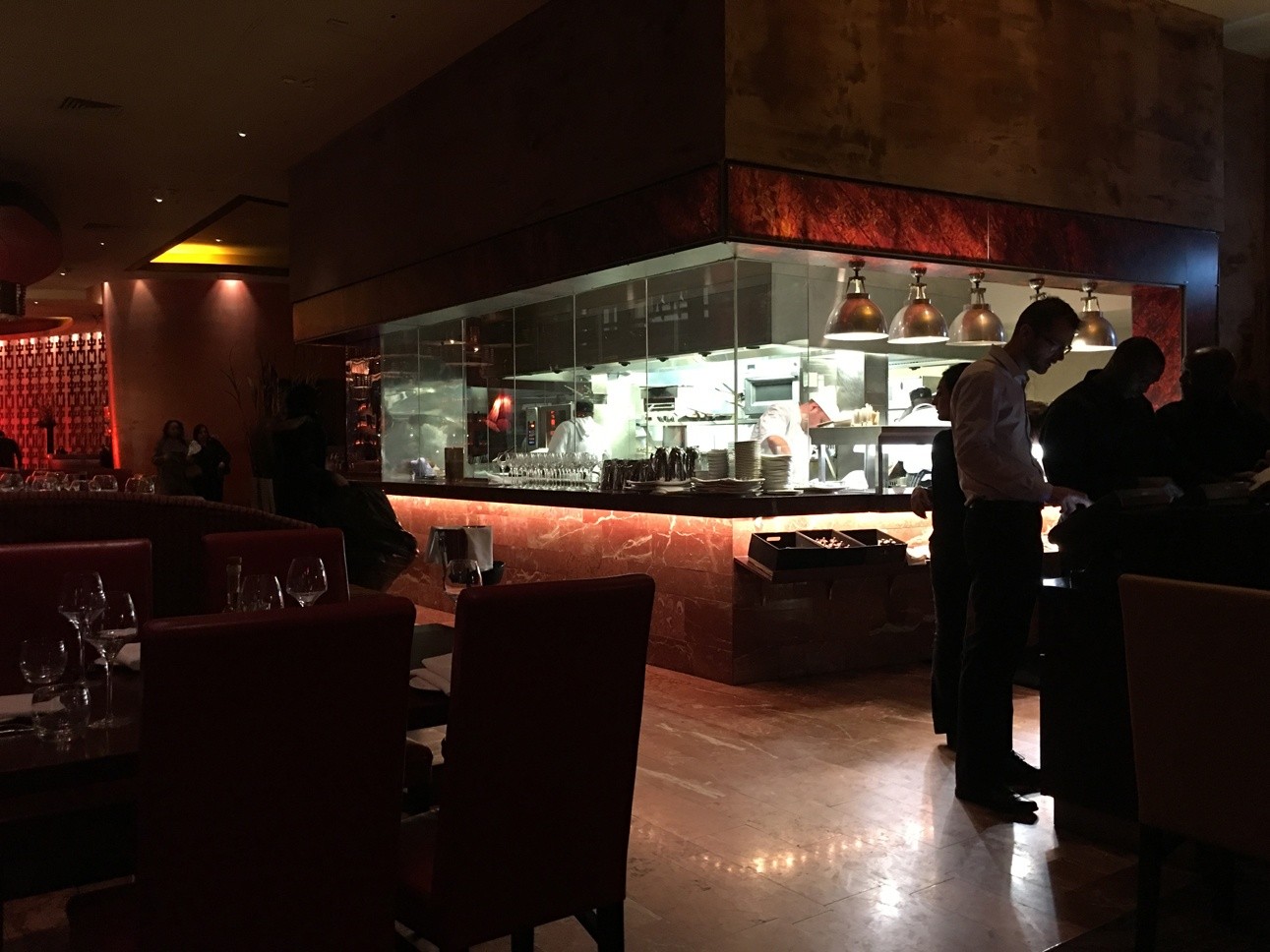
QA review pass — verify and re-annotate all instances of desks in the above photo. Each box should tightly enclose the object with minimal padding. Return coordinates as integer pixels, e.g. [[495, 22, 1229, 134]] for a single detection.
[[1, 623, 454, 797]]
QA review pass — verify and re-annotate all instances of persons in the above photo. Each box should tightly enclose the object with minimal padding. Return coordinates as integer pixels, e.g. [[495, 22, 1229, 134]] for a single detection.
[[306, 471, 417, 589], [1037, 337, 1166, 805], [1155, 346, 1266, 585], [182, 424, 231, 503], [911, 363, 1047, 753], [0, 431, 22, 468], [951, 299, 1094, 813], [152, 421, 193, 493]]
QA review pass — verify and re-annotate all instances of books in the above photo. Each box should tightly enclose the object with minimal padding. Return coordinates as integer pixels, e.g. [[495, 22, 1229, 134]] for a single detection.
[[1194, 465, 1270, 497], [1116, 477, 1175, 506]]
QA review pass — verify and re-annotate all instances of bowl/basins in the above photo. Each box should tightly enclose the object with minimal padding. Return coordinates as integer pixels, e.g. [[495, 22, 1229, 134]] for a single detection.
[[694, 441, 792, 489]]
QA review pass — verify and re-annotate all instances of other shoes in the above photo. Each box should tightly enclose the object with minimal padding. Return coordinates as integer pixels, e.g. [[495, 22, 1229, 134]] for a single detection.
[[1006, 751, 1043, 794], [956, 781, 1039, 814], [947, 728, 957, 752]]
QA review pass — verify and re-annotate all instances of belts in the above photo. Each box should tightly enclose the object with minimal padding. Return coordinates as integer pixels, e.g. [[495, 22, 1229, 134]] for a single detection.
[[967, 498, 1044, 512]]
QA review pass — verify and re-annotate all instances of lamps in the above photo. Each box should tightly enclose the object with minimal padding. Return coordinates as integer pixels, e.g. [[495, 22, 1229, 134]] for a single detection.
[[947, 272, 1008, 346], [1072, 282, 1117, 352], [824, 260, 889, 340], [888, 266, 949, 344], [484, 393, 513, 432]]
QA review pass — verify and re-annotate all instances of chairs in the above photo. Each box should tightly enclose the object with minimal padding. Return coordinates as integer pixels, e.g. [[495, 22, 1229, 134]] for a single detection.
[[394, 574, 656, 951], [65, 596, 415, 952], [201, 528, 434, 810], [1118, 573, 1270, 952], [0, 539, 154, 696]]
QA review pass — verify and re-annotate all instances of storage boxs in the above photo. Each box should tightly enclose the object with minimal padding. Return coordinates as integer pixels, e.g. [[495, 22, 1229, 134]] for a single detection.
[[747, 528, 908, 570]]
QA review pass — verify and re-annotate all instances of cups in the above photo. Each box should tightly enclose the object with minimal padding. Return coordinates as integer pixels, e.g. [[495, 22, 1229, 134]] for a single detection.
[[19, 635, 69, 684], [236, 572, 284, 613], [0, 470, 118, 492], [124, 477, 155, 494]]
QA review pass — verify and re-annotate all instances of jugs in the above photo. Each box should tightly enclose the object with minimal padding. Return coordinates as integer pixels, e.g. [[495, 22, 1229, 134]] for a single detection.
[[599, 445, 700, 492]]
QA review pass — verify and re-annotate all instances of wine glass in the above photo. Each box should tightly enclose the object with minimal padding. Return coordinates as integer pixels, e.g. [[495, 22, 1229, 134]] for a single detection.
[[80, 590, 139, 730], [57, 570, 107, 707], [498, 452, 599, 493], [286, 557, 328, 608], [32, 681, 92, 764], [443, 558, 483, 605]]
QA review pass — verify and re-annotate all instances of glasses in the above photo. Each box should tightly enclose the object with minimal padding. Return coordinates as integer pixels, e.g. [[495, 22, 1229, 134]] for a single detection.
[[1027, 323, 1071, 356]]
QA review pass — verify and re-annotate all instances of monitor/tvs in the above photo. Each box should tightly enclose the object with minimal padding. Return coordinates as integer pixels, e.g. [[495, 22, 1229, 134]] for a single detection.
[[1048, 486, 1176, 544], [1191, 481, 1252, 511]]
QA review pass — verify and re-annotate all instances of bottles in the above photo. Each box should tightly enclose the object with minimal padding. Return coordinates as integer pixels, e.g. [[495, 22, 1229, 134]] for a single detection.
[[221, 555, 244, 615], [133, 473, 144, 493]]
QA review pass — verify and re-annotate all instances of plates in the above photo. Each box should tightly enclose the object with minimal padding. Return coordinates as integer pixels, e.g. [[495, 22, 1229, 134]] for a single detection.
[[409, 677, 442, 691], [623, 477, 847, 494]]
